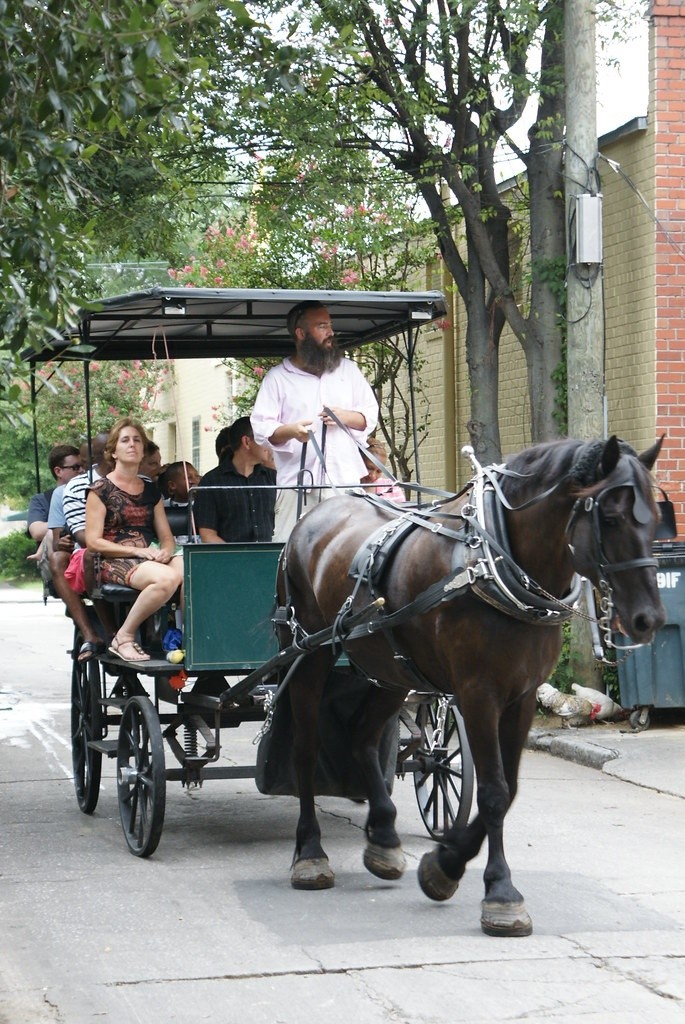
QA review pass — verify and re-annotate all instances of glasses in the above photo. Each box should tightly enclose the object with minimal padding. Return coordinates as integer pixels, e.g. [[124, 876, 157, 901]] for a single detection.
[[60, 463, 84, 471]]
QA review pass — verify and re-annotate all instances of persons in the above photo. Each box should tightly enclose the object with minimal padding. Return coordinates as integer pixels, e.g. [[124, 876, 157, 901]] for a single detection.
[[23, 416, 408, 666], [249, 301, 379, 544]]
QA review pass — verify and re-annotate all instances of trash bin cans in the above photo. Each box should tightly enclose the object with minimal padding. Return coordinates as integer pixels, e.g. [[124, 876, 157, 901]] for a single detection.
[[610, 554, 684, 731]]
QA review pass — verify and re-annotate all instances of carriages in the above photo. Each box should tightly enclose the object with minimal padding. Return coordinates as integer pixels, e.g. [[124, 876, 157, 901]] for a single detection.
[[19, 284, 676, 937]]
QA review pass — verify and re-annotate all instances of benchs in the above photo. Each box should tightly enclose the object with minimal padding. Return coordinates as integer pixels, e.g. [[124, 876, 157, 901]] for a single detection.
[[90, 548, 139, 605]]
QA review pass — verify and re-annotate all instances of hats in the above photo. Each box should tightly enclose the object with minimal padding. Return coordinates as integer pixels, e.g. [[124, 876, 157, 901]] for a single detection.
[[286, 300, 322, 338]]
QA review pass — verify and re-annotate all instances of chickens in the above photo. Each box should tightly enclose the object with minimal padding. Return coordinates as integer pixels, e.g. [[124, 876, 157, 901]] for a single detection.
[[570, 683, 626, 727], [535, 682, 602, 732]]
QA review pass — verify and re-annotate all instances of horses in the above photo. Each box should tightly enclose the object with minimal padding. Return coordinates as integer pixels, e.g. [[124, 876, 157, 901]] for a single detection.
[[272, 432, 669, 938]]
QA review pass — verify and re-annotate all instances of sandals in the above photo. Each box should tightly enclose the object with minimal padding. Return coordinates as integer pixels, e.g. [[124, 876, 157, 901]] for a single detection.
[[109, 635, 150, 661]]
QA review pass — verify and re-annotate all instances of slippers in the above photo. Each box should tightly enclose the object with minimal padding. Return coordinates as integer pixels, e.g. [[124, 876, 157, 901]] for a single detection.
[[80, 641, 106, 663]]
